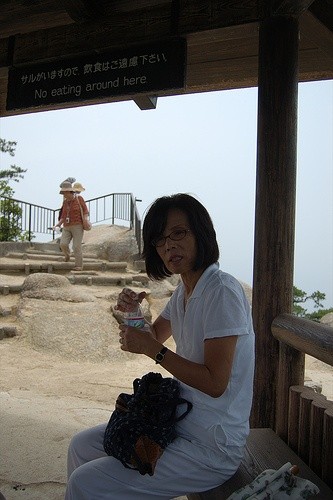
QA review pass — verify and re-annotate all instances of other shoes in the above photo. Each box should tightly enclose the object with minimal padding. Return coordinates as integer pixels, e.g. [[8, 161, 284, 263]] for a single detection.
[[73, 267, 82, 271], [64, 257, 70, 262]]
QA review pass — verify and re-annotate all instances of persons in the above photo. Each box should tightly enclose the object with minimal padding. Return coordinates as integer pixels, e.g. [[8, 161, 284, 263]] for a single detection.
[[53, 178, 92, 270], [66, 193, 255, 500]]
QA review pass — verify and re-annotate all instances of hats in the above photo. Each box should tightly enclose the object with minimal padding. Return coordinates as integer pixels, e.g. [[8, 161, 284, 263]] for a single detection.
[[73, 183, 85, 192], [60, 182, 75, 194]]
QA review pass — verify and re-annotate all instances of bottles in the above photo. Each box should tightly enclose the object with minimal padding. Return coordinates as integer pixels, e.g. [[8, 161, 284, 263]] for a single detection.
[[52, 226, 62, 233]]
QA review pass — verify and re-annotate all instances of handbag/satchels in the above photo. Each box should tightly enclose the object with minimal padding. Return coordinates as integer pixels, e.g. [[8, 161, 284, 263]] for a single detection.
[[104, 372, 193, 476], [82, 218, 92, 231]]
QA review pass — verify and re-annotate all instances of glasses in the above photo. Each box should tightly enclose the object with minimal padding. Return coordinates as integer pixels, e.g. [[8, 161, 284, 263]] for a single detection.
[[152, 229, 191, 247]]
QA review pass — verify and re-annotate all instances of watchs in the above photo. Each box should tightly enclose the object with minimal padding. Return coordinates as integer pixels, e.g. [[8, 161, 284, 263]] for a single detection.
[[155, 347, 168, 364]]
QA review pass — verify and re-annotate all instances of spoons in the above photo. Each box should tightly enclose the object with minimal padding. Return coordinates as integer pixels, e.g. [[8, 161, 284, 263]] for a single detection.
[[125, 284, 153, 326]]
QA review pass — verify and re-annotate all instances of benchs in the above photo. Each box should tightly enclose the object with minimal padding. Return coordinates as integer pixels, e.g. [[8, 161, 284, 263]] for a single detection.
[[188, 428, 333, 500]]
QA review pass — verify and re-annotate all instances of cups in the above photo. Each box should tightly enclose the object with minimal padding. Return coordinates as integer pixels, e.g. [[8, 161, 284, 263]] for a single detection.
[[122, 316, 145, 329]]
